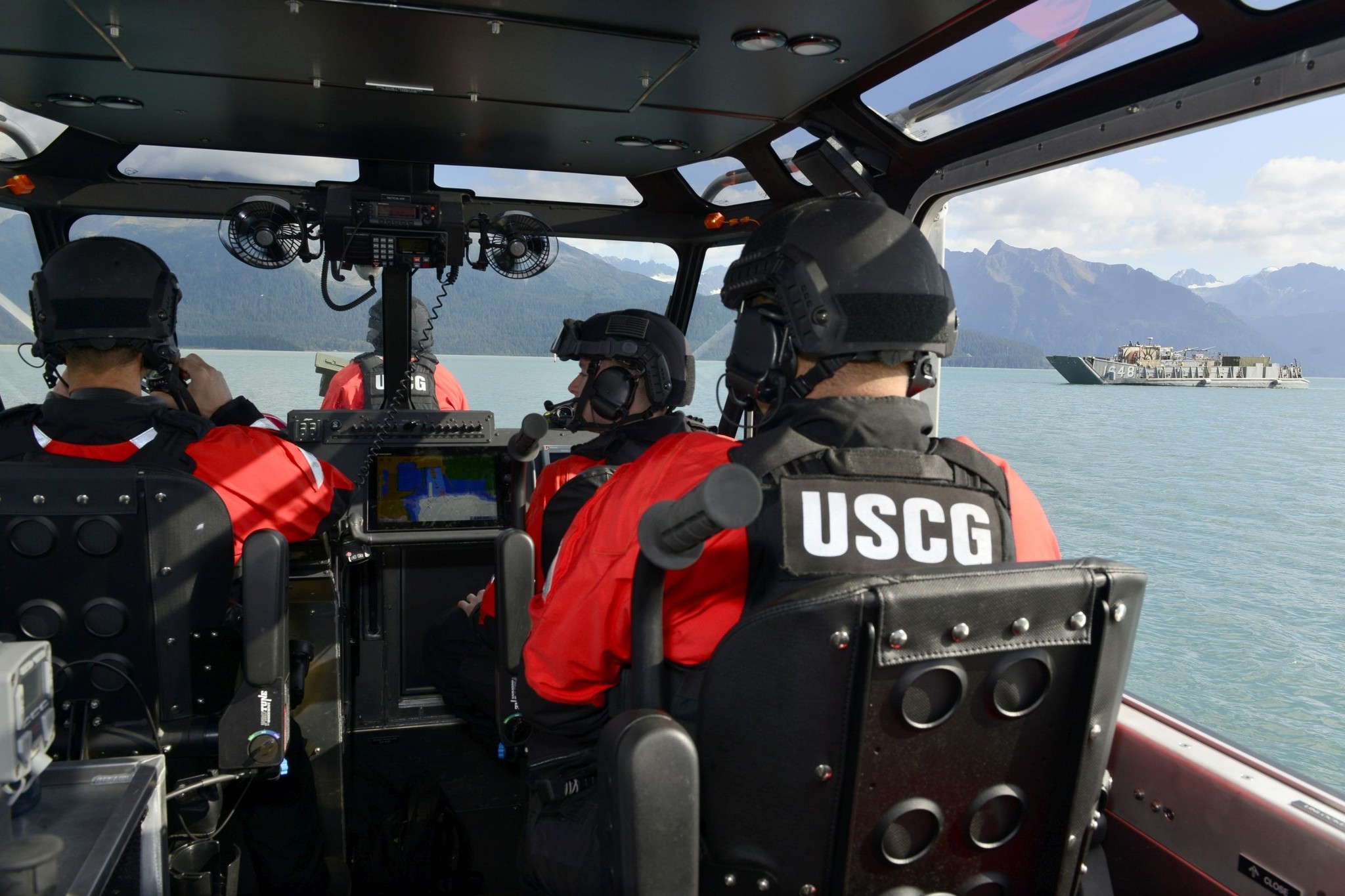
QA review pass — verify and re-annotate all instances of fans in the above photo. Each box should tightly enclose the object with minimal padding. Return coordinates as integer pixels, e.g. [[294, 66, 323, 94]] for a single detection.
[[218, 194, 304, 268], [479, 209, 560, 279]]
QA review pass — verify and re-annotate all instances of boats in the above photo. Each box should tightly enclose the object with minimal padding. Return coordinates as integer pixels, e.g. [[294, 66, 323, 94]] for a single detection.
[[1045, 337, 1310, 389]]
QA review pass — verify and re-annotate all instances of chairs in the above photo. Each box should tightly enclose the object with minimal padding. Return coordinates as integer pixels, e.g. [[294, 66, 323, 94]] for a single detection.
[[495, 465, 619, 761], [605, 554, 1149, 896], [0, 461, 290, 782]]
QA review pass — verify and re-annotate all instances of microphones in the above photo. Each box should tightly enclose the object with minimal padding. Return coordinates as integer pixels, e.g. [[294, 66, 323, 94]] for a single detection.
[[543, 396, 580, 411]]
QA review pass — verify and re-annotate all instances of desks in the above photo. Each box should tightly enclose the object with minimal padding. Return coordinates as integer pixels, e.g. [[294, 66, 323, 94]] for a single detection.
[[0, 754, 167, 896]]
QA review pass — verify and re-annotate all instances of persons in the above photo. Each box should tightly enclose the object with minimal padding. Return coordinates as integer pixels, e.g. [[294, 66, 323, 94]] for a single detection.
[[319, 298, 467, 414], [0, 237, 340, 896], [514, 187, 1065, 896], [434, 309, 723, 764]]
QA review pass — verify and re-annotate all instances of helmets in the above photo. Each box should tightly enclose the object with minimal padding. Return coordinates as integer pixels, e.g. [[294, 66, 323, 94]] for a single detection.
[[551, 309, 694, 406], [719, 191, 960, 361], [27, 237, 181, 355], [367, 294, 433, 350]]
